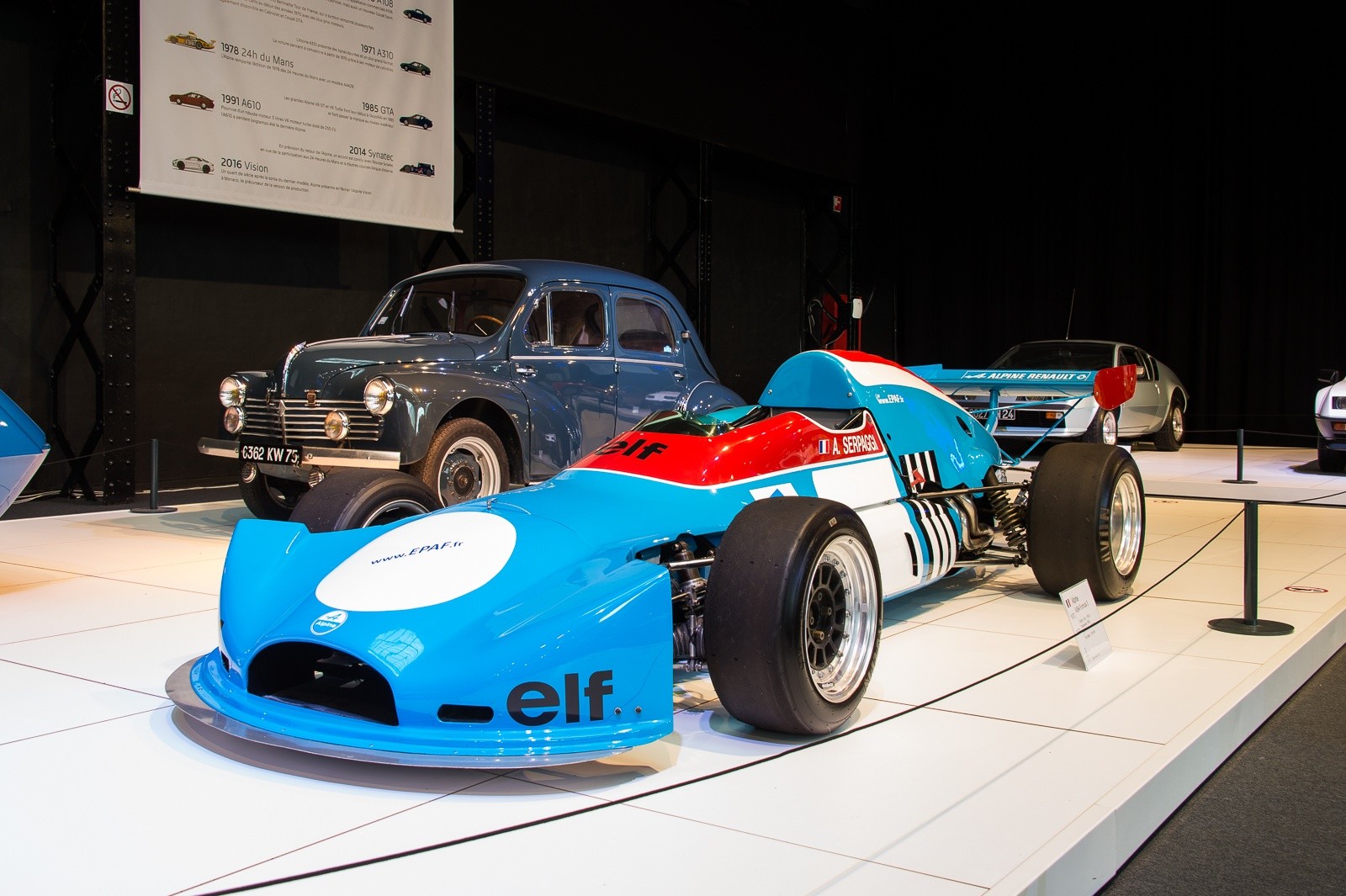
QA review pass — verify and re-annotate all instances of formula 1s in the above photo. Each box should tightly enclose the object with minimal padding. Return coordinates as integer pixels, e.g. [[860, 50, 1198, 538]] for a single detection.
[[162, 332, 1147, 768]]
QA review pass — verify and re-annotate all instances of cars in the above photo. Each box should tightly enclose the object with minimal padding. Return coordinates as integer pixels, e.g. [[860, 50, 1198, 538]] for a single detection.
[[400, 162, 435, 177], [404, 8, 431, 23], [1312, 369, 1346, 470], [955, 340, 1187, 451], [173, 156, 214, 175], [198, 260, 751, 522], [169, 92, 215, 110], [400, 114, 432, 130], [400, 61, 430, 76], [165, 30, 217, 50]]
